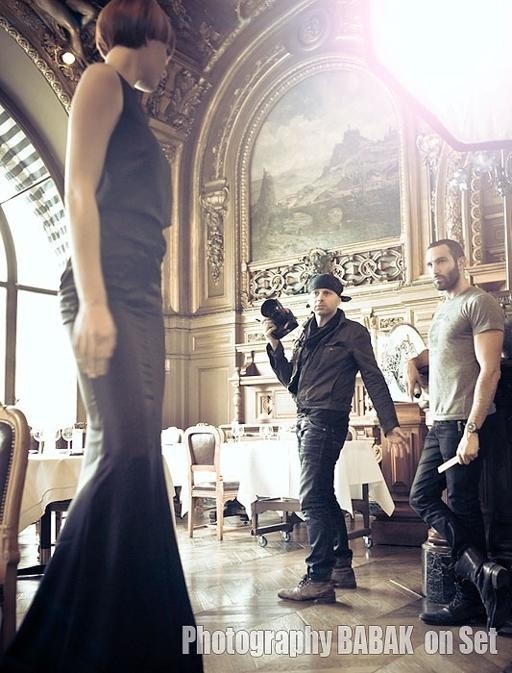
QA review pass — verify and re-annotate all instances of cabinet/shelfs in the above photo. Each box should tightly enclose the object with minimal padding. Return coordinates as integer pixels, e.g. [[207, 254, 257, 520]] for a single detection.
[[373, 403, 435, 547]]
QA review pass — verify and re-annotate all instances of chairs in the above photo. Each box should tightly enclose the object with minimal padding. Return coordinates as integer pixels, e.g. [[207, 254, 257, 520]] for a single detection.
[[0, 402, 30, 656]]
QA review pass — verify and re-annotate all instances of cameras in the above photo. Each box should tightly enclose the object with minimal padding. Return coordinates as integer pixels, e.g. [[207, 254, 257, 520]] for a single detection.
[[260, 298, 299, 339]]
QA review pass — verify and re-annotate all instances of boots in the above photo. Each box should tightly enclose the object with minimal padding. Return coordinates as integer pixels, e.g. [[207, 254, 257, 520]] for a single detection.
[[419, 548, 510, 631]]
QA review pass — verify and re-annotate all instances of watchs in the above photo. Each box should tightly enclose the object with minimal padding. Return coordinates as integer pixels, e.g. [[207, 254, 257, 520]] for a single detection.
[[463, 423, 478, 434]]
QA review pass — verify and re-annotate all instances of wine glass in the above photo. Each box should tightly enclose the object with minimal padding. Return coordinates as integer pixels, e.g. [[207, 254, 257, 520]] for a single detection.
[[32, 424, 75, 452], [231, 424, 291, 443]]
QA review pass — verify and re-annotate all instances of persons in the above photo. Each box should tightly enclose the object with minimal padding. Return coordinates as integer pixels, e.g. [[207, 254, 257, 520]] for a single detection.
[[408, 239, 509, 635], [260, 276, 406, 606], [0, 1, 207, 673]]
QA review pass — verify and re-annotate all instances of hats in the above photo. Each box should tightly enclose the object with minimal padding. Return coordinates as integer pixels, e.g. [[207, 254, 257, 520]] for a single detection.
[[308, 274, 352, 301]]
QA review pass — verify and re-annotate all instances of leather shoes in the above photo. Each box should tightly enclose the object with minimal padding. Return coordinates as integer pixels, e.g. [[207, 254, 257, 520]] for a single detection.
[[277, 567, 355, 603]]
